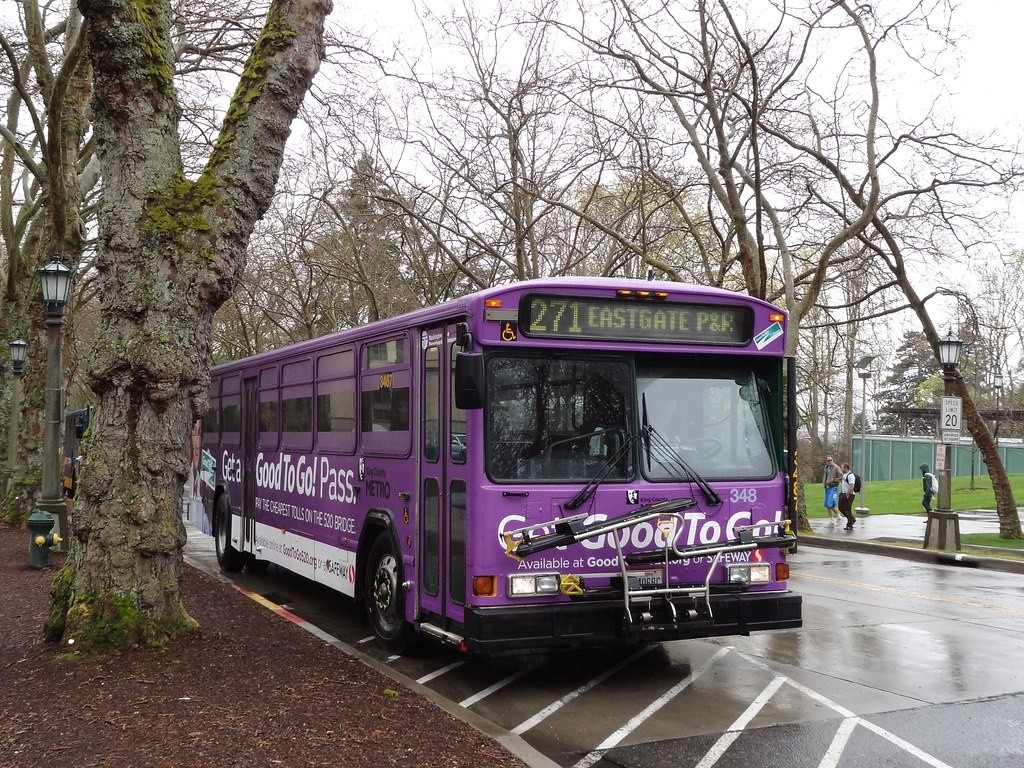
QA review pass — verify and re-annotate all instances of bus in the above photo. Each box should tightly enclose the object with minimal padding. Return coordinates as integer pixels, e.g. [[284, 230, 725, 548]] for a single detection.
[[185, 274, 803, 662]]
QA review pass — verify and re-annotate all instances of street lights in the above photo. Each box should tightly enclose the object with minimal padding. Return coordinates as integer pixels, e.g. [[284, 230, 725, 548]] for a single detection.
[[854, 355, 876, 514], [36, 249, 72, 556], [934, 326, 965, 511]]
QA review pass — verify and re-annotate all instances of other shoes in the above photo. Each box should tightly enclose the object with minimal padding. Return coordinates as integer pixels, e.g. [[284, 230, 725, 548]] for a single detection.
[[836, 516, 844, 526], [847, 517, 856, 526], [824, 522, 835, 528], [924, 520, 928, 522], [844, 526, 853, 530]]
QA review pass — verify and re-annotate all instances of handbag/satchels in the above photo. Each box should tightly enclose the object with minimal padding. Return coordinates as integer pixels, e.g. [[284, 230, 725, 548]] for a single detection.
[[925, 473, 938, 494]]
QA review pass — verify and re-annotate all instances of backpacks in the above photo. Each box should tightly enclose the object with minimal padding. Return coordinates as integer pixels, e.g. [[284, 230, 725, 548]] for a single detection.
[[846, 472, 861, 493]]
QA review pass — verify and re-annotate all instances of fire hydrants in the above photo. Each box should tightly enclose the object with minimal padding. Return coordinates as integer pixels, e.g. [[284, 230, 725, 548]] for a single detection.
[[26, 511, 65, 572]]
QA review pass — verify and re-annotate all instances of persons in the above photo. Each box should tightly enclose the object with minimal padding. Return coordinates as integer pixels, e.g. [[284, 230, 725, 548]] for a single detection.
[[838, 463, 856, 530], [189, 414, 212, 535], [648, 399, 677, 446], [919, 463, 934, 523], [823, 455, 844, 527]]
